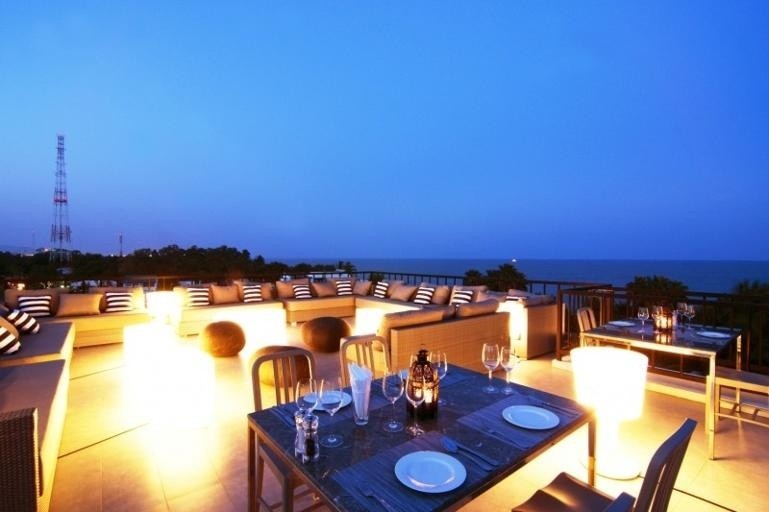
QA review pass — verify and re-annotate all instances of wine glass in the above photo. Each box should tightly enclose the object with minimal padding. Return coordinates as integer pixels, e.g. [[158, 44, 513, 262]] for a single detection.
[[428, 350, 449, 408], [480, 340, 500, 396], [319, 376, 347, 449], [651, 305, 664, 335], [381, 369, 406, 434], [404, 374, 428, 437], [676, 301, 687, 328], [409, 349, 428, 368], [500, 344, 519, 397], [684, 305, 697, 331], [637, 307, 649, 332], [294, 376, 319, 412]]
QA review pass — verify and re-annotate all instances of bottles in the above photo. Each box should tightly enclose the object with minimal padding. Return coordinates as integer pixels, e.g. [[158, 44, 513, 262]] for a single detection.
[[292, 405, 321, 466]]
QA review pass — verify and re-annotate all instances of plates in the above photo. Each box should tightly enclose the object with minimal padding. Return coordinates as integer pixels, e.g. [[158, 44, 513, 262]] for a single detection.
[[609, 320, 635, 327], [303, 388, 353, 409], [697, 330, 731, 338], [394, 449, 467, 493], [397, 367, 409, 381], [499, 404, 562, 432]]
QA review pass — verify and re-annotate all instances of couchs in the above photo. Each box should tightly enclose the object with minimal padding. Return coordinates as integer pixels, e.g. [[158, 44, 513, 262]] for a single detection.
[[0, 358, 69, 512], [352, 299, 513, 384]]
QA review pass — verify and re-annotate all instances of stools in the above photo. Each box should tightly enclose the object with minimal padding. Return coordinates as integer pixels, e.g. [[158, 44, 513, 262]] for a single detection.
[[704, 365, 769, 462]]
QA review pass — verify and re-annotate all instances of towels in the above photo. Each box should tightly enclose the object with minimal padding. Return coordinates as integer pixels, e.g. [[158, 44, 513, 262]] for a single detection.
[[347, 364, 374, 420]]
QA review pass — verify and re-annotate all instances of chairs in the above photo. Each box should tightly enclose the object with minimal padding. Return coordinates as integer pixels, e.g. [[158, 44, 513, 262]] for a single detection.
[[248, 344, 339, 511], [598, 490, 640, 511], [509, 416, 699, 510], [575, 305, 599, 348], [338, 335, 393, 391]]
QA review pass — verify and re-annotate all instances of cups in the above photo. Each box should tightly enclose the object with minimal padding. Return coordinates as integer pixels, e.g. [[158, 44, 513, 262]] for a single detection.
[[349, 376, 373, 426]]
